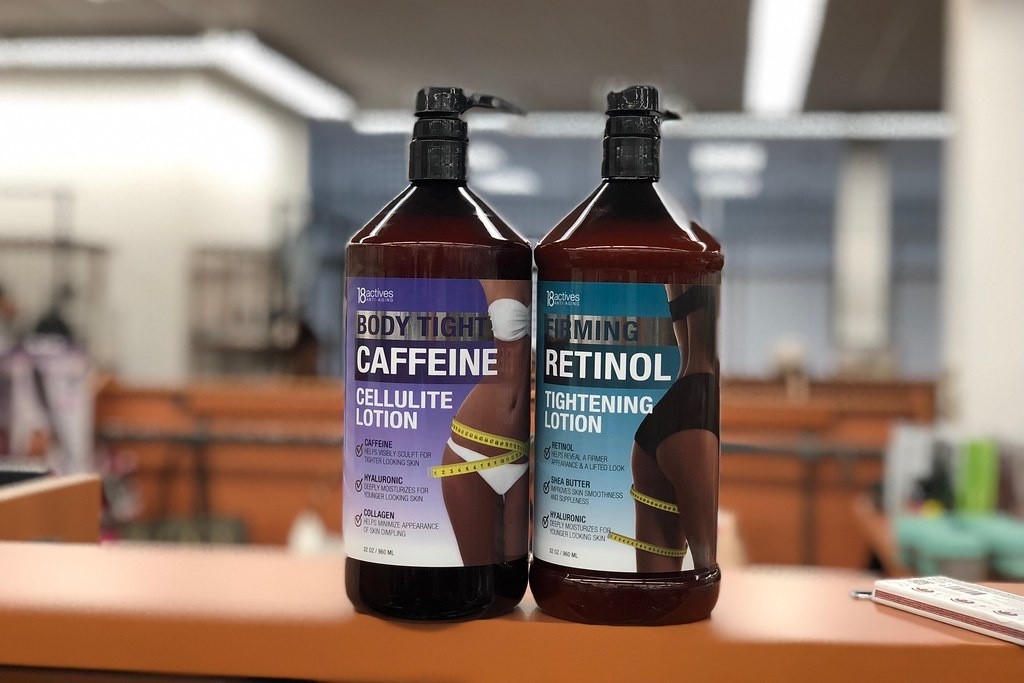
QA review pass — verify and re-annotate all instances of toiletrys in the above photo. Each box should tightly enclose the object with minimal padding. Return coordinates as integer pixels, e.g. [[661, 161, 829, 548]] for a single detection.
[[341, 86, 532, 626], [530, 82, 724, 624]]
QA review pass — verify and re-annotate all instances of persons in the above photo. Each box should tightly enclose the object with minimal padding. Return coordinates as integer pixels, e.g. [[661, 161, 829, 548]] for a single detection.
[[440, 280, 527, 565], [0, 286, 105, 484], [631, 283, 716, 574]]
